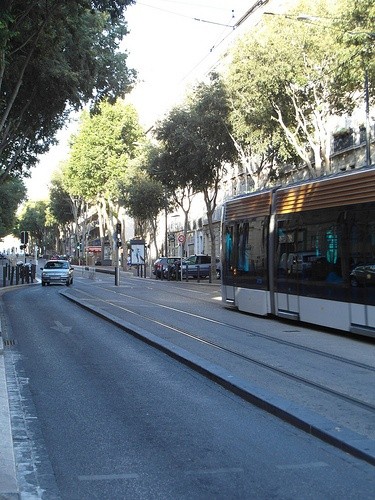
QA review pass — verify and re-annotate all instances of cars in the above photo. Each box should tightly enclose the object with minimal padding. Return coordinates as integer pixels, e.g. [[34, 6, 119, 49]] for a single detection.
[[49, 252, 70, 259], [349, 265, 375, 287], [40, 260, 75, 286]]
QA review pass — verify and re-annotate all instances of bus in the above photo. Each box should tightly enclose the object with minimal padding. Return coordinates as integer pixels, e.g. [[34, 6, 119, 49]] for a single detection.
[[219, 164, 375, 339]]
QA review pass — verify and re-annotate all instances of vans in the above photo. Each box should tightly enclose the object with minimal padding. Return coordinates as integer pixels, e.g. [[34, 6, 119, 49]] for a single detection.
[[279, 252, 322, 279]]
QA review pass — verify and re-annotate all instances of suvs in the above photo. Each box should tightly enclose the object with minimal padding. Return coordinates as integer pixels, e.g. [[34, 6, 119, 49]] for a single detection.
[[153, 254, 221, 281]]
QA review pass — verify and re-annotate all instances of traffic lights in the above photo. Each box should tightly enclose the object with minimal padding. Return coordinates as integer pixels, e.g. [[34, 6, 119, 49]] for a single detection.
[[21, 231, 29, 244]]
